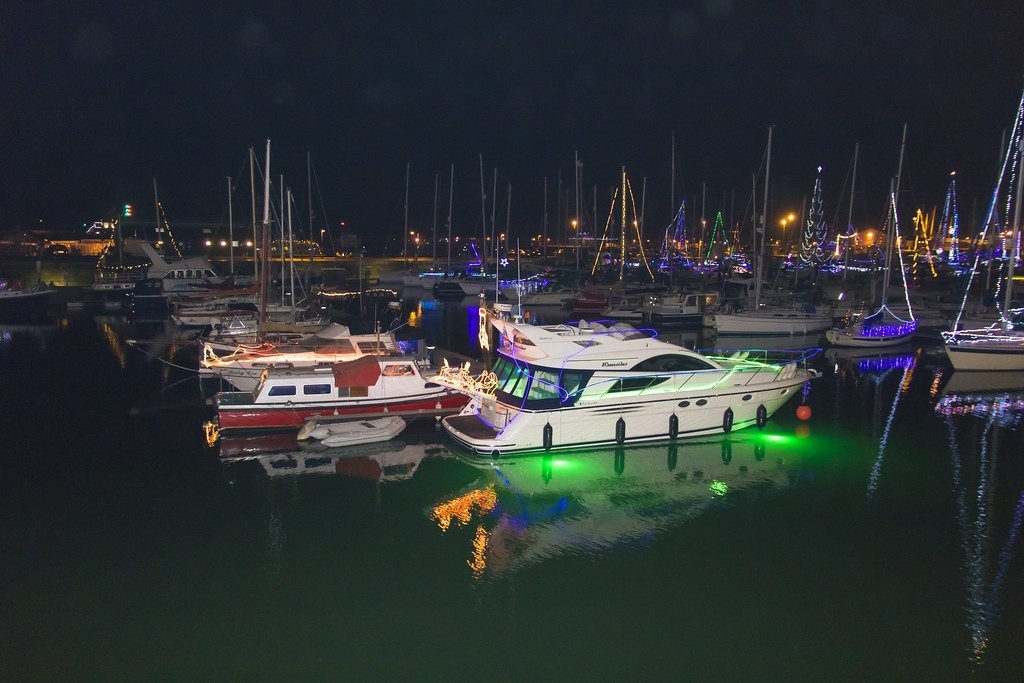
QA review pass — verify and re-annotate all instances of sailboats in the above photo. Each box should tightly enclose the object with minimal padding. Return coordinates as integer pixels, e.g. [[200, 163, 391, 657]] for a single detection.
[[934, 371, 1024, 531], [91, 97, 1024, 371]]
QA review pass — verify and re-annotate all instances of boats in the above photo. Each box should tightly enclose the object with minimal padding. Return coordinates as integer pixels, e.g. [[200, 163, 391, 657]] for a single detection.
[[202, 320, 481, 448], [219, 436, 457, 508], [443, 239, 823, 456]]
